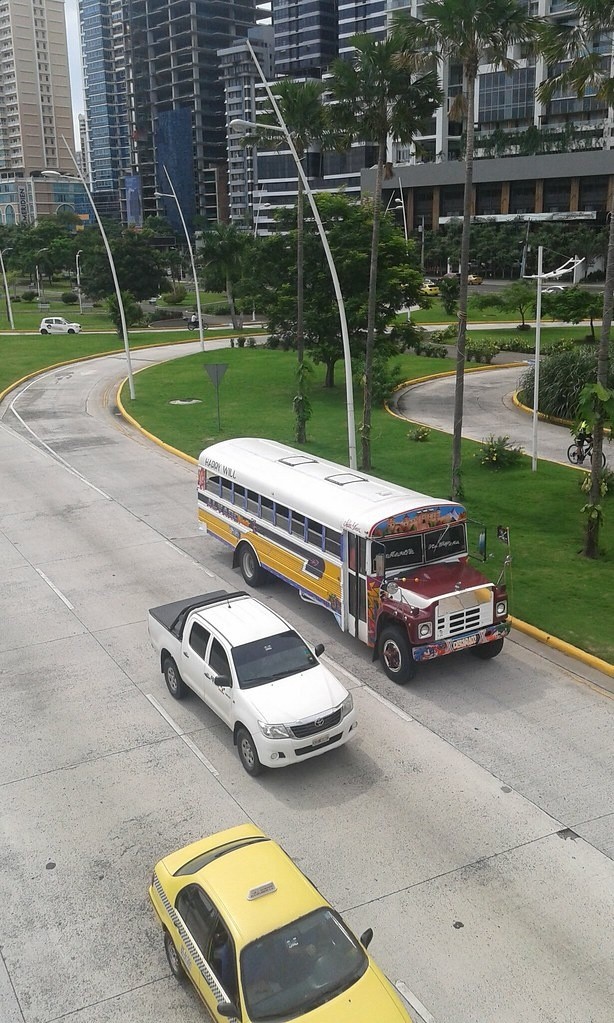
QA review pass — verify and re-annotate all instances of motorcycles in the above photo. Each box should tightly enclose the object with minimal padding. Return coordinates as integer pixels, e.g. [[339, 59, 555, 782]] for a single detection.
[[187, 319, 209, 331]]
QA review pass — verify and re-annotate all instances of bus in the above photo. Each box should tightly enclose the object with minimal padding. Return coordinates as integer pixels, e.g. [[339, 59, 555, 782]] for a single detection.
[[196, 437, 513, 684]]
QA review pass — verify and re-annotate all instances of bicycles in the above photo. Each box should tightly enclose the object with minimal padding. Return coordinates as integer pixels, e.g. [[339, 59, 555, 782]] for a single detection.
[[567, 441, 606, 469]]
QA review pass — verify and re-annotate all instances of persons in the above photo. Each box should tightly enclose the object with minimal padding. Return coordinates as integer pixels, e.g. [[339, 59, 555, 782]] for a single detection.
[[191, 313, 198, 328], [576, 420, 593, 465]]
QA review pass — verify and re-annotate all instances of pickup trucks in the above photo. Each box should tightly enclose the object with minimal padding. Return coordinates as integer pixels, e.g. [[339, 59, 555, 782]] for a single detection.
[[147, 590, 360, 778]]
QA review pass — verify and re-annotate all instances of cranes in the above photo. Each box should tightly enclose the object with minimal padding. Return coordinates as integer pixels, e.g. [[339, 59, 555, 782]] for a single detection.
[[443, 273, 460, 285]]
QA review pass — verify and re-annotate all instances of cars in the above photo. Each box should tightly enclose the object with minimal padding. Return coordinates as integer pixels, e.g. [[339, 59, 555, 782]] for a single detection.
[[148, 294, 162, 305], [400, 276, 445, 291], [148, 823, 414, 1023], [541, 285, 570, 295], [467, 274, 483, 286], [417, 281, 440, 297], [39, 317, 82, 336]]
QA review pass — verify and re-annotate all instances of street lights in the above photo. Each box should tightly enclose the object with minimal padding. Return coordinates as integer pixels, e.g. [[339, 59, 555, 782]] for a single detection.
[[36, 248, 49, 304], [153, 165, 205, 352], [229, 38, 357, 472], [41, 133, 136, 401], [0, 248, 16, 331], [75, 250, 84, 314], [395, 176, 410, 322]]
